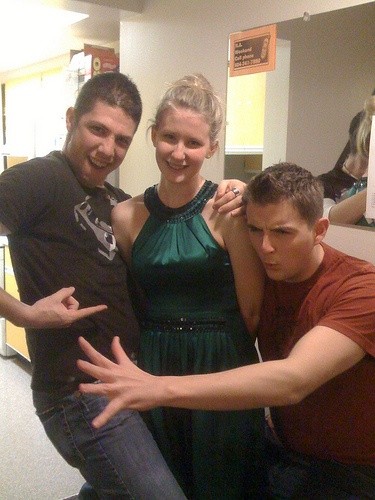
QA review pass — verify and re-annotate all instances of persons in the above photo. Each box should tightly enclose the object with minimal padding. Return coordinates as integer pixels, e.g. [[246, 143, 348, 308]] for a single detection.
[[317, 88, 375, 230], [76, 163, 375, 500], [0, 72, 250, 500], [110, 72, 266, 500]]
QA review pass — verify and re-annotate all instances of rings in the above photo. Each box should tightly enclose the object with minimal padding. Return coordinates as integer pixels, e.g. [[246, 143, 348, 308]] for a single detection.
[[232, 186, 241, 198]]
